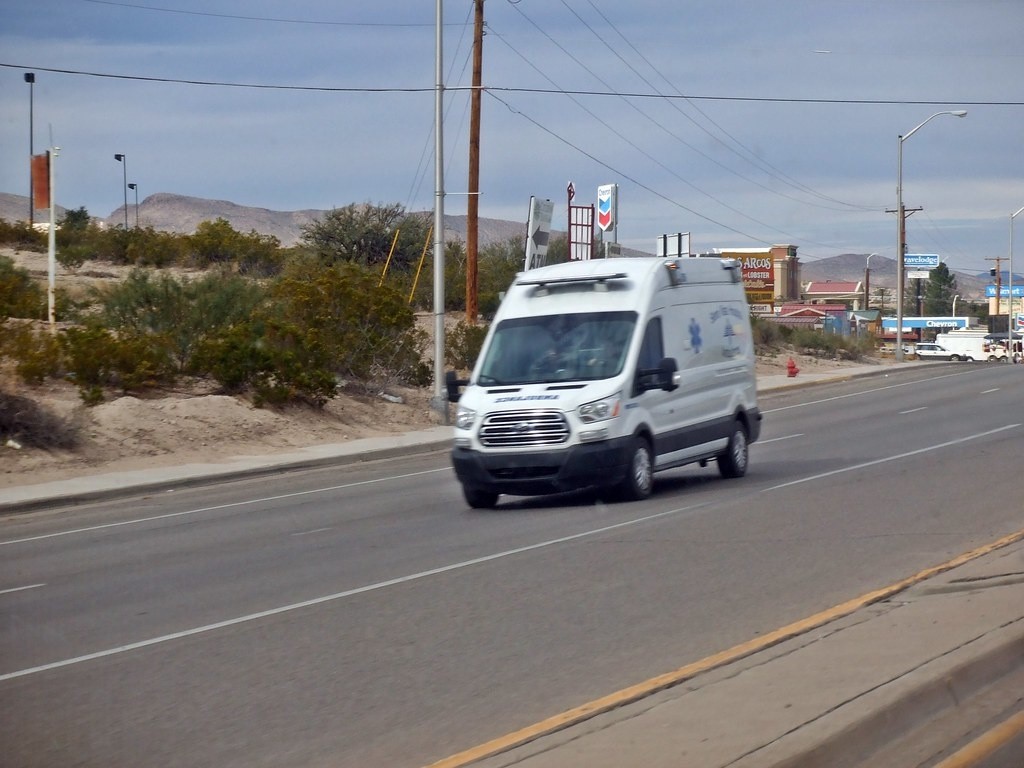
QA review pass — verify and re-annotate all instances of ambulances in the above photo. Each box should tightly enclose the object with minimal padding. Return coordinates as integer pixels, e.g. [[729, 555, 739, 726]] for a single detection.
[[444, 257, 763, 509]]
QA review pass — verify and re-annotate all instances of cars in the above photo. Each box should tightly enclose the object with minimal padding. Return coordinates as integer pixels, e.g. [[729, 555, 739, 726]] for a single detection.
[[915, 342, 967, 362], [875, 347, 887, 353], [889, 347, 897, 355], [904, 346, 917, 355]]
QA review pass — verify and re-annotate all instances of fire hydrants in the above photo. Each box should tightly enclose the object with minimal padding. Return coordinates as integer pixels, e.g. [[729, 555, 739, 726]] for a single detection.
[[787, 357, 800, 378]]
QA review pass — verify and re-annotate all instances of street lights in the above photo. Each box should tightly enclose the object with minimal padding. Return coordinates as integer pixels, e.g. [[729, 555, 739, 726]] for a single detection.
[[23, 71, 35, 230], [128, 182, 139, 230], [115, 154, 128, 232], [879, 288, 886, 316], [865, 253, 878, 310], [897, 110, 966, 355], [952, 294, 959, 329]]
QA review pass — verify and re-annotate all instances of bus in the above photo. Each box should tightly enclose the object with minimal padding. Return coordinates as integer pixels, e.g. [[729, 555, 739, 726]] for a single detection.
[[935, 333, 993, 362]]
